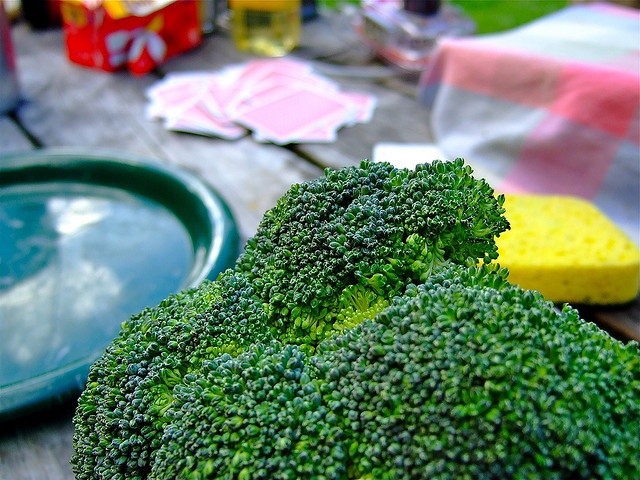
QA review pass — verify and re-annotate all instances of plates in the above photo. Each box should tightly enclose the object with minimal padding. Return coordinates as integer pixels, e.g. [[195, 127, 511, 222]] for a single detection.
[[1, 152, 240, 417]]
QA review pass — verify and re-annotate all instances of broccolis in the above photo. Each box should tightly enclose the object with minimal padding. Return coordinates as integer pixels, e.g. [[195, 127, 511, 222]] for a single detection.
[[68, 154, 640, 479]]
